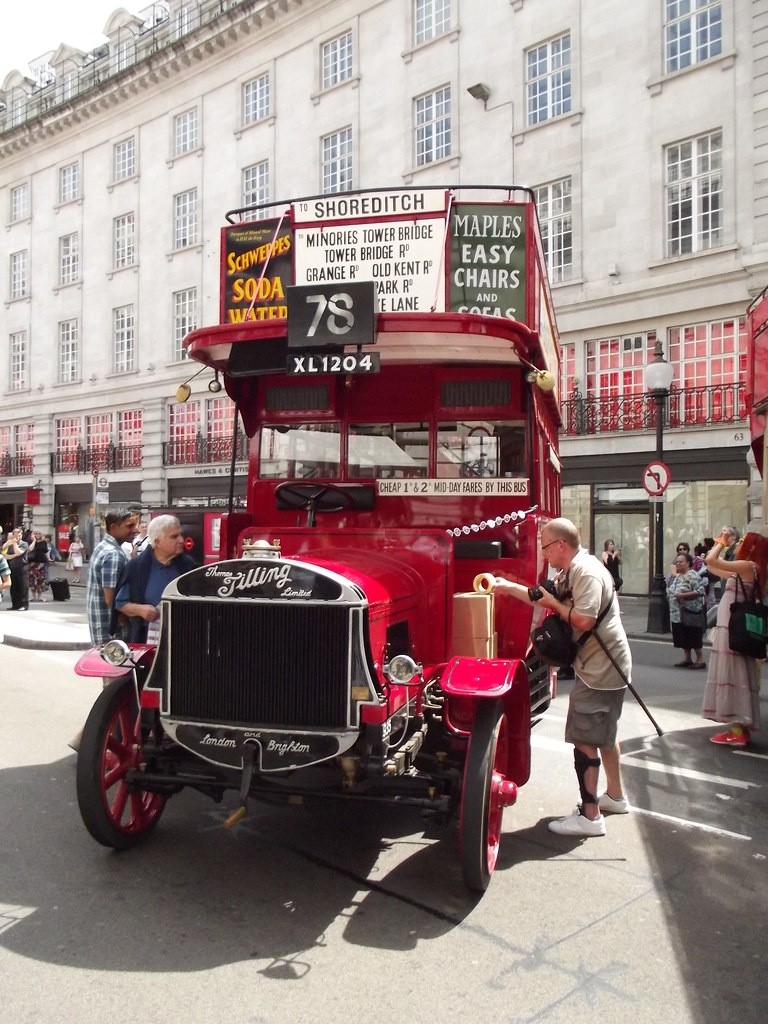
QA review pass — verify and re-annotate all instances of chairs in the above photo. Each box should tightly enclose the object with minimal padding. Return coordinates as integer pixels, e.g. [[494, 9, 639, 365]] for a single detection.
[[272, 482, 381, 529]]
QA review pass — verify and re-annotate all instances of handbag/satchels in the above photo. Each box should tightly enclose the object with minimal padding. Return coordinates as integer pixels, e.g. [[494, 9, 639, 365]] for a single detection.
[[531, 615, 576, 669], [728, 561, 768, 659], [66, 559, 74, 571], [616, 578, 623, 586], [679, 606, 705, 628]]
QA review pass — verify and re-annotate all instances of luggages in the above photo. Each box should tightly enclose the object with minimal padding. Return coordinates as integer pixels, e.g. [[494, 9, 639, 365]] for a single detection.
[[51, 577, 70, 601]]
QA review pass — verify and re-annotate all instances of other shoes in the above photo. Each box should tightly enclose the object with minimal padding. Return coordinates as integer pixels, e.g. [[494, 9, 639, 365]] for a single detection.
[[71, 580, 76, 583], [69, 731, 82, 751], [30, 598, 37, 601], [7, 607, 15, 610], [18, 607, 26, 611], [75, 580, 80, 583], [674, 661, 693, 667], [38, 597, 46, 602], [688, 662, 705, 669]]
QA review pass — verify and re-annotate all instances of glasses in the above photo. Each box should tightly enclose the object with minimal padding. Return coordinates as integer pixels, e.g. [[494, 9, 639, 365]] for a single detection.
[[679, 548, 686, 551], [541, 539, 566, 551], [739, 538, 744, 544]]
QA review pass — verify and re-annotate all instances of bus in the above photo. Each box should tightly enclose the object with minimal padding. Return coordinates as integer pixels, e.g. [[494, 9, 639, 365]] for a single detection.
[[70, 184, 574, 895]]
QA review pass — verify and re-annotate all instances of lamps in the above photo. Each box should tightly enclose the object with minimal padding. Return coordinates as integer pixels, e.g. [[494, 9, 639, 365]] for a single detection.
[[608, 264, 619, 277], [467, 82, 492, 100]]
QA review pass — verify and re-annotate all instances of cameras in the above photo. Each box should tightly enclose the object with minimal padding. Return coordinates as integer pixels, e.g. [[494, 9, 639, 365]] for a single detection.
[[527, 580, 557, 602]]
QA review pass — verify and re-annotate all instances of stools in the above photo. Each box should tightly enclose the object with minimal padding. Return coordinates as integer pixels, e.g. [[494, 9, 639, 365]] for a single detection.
[[454, 540, 503, 560]]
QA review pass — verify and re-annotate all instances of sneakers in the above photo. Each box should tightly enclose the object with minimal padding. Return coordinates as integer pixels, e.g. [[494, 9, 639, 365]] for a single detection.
[[710, 730, 747, 746], [599, 792, 628, 814], [549, 802, 606, 836]]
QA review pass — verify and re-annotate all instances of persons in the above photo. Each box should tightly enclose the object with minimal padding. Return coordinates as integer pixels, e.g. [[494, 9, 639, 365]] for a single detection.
[[720, 526, 740, 600], [68, 508, 142, 753], [121, 535, 139, 565], [115, 514, 200, 644], [132, 521, 151, 557], [68, 535, 85, 583], [0, 524, 62, 611], [491, 517, 632, 836], [665, 537, 720, 670], [706, 528, 768, 746], [602, 539, 625, 615]]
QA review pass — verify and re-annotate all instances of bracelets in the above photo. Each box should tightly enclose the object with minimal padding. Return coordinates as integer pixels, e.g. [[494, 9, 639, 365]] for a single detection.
[[714, 537, 729, 547]]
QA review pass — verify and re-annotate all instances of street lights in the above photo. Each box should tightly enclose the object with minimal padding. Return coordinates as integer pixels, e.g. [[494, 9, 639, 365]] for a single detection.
[[641, 339, 671, 634]]
